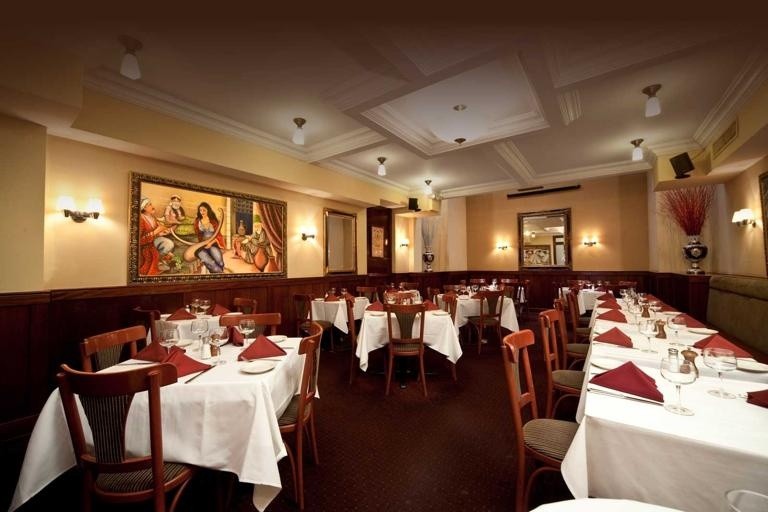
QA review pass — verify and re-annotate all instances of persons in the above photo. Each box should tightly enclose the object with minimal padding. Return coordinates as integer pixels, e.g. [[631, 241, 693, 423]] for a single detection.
[[161, 194, 187, 224], [193, 201, 227, 274], [241, 213, 275, 264], [139, 197, 174, 271]]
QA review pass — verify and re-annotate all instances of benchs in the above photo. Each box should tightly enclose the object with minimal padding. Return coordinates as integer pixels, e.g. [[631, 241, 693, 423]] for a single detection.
[[702, 275, 768, 365]]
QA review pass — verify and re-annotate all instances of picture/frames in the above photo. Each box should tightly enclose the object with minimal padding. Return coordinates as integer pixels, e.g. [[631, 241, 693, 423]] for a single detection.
[[127, 171, 287, 287], [759, 170, 768, 276], [523, 244, 551, 266]]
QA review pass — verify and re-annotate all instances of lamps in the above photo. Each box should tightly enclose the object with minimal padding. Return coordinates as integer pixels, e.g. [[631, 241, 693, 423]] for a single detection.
[[732, 208, 755, 227], [584, 232, 596, 246], [498, 240, 509, 249], [57, 196, 104, 223], [377, 157, 386, 176], [118, 35, 143, 81], [631, 139, 643, 160], [400, 238, 409, 247], [430, 105, 488, 145], [642, 84, 661, 117], [424, 180, 432, 193], [291, 117, 305, 145]]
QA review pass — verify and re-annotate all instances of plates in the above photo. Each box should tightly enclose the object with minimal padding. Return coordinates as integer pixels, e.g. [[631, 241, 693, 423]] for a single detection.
[[735, 358, 767, 372], [267, 335, 287, 343], [590, 358, 626, 370], [688, 327, 719, 335], [239, 361, 275, 374], [173, 338, 192, 346]]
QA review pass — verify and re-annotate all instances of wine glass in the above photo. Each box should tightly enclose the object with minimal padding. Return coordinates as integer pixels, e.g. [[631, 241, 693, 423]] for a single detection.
[[210, 329, 229, 364], [191, 321, 209, 350], [191, 299, 211, 319], [639, 321, 659, 354], [575, 278, 610, 291], [660, 358, 698, 417], [324, 287, 349, 300], [238, 320, 256, 348], [619, 286, 683, 321], [702, 348, 735, 400], [667, 316, 686, 346], [159, 327, 179, 354]]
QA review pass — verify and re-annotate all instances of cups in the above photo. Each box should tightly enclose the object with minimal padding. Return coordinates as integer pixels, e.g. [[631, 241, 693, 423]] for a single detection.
[[455, 278, 499, 298], [390, 281, 409, 292]]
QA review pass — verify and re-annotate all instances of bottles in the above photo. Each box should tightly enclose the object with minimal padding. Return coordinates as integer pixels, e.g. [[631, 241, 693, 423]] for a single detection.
[[667, 348, 680, 372], [185, 304, 190, 314], [680, 348, 699, 378]]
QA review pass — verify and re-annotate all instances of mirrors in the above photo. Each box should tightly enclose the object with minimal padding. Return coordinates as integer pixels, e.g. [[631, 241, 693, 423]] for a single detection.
[[323, 207, 357, 273], [517, 208, 571, 273]]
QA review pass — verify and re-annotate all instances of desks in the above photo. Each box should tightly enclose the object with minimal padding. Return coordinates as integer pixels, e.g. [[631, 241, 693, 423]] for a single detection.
[[559, 291, 768, 512]]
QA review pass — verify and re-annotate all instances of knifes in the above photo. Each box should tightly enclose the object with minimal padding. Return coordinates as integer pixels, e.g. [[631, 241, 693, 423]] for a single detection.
[[184, 363, 217, 385]]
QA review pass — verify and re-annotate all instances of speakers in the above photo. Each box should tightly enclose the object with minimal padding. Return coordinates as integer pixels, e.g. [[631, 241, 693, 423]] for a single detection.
[[670, 151, 695, 176], [408, 198, 417, 210]]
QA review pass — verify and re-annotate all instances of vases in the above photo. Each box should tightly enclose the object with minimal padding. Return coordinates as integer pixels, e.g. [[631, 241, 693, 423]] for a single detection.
[[683, 235, 707, 275], [422, 246, 434, 273]]
